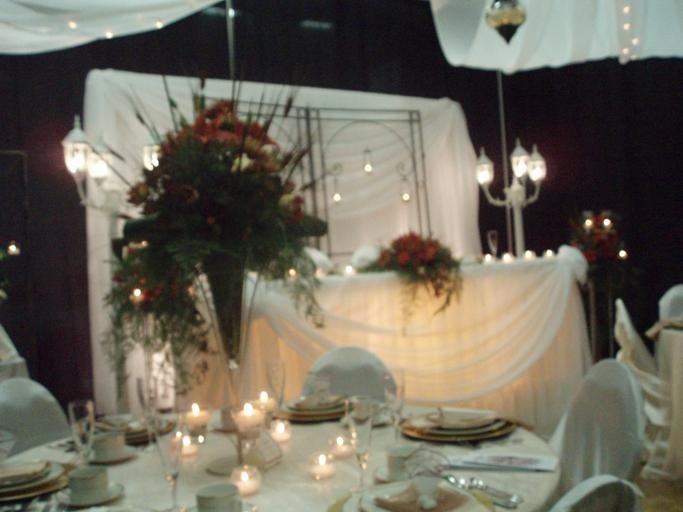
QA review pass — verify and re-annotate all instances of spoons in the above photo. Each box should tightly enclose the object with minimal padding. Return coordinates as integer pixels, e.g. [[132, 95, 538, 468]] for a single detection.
[[447, 474, 517, 509], [470, 475, 524, 503]]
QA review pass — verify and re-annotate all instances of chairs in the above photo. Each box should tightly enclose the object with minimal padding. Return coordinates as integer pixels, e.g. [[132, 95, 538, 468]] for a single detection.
[[614, 299, 666, 478], [549, 359, 646, 503], [552, 474, 639, 512], [1, 377, 69, 457], [301, 346, 397, 405], [660, 285, 682, 320]]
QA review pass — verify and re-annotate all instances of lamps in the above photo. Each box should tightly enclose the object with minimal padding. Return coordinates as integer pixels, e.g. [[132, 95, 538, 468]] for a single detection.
[[486, 0, 526, 43]]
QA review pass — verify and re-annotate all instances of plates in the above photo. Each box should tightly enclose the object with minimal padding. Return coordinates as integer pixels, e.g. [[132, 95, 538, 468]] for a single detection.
[[86, 412, 155, 434], [373, 464, 390, 482], [327, 480, 496, 512], [406, 410, 506, 436], [56, 483, 126, 507], [358, 479, 479, 511], [125, 417, 177, 444], [0, 472, 70, 503], [399, 417, 517, 443], [0, 463, 72, 492], [273, 404, 348, 422], [1, 460, 49, 487], [280, 396, 351, 416], [91, 426, 152, 438], [79, 446, 136, 463], [184, 501, 260, 512]]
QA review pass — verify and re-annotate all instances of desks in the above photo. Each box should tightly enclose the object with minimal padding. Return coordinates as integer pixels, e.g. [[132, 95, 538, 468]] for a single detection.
[[656, 316, 682, 478], [172, 257, 589, 433], [0, 404, 563, 512]]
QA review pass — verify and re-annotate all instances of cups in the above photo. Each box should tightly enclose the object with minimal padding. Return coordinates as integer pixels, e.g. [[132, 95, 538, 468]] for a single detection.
[[385, 446, 416, 474], [195, 484, 241, 512], [90, 431, 125, 456], [69, 467, 109, 501]]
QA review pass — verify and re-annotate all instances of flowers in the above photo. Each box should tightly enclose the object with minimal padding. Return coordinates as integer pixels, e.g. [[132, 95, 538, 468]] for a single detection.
[[357, 228, 464, 318], [564, 208, 628, 274], [83, 49, 343, 395]]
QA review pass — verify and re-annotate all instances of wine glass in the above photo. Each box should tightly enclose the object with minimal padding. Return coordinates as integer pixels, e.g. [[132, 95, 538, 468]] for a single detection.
[[487, 228, 499, 263], [346, 395, 375, 491], [154, 407, 190, 512], [68, 400, 95, 466], [267, 361, 287, 406], [383, 366, 408, 441]]
